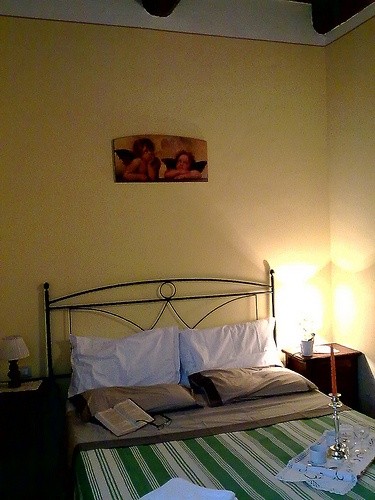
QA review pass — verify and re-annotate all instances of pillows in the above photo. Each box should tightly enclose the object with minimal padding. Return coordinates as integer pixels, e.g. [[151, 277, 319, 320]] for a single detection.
[[66, 324, 181, 396], [68, 383, 206, 428], [187, 365, 319, 408], [179, 317, 284, 388]]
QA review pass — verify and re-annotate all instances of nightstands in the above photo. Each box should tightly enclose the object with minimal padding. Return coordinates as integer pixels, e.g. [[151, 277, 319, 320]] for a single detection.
[[0, 377, 51, 464], [281, 342, 362, 409]]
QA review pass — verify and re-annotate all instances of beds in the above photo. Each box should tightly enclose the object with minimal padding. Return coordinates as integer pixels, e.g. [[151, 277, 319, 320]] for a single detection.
[[42, 268, 375, 500]]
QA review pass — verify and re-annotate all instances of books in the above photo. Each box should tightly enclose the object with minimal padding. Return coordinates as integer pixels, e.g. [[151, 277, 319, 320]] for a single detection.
[[95, 398, 154, 438]]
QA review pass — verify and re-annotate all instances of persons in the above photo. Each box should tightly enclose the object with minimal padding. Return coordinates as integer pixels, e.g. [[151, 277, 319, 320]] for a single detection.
[[123, 138, 156, 181], [165, 151, 202, 178]]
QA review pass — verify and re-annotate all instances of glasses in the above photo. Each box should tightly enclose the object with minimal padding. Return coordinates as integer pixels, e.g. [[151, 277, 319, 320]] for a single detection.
[[136, 413, 172, 430]]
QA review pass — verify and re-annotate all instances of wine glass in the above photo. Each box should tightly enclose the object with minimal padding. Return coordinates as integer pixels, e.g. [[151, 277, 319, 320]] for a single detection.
[[353, 421, 370, 455]]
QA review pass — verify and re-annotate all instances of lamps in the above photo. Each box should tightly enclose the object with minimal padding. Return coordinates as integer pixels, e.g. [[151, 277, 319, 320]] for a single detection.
[[0, 334, 31, 389]]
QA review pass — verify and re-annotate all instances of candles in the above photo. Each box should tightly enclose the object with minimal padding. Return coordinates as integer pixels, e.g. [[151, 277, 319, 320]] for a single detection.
[[329, 344, 338, 394]]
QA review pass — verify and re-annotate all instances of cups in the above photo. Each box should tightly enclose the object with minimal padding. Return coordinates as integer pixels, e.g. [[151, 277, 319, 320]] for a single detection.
[[326, 431, 336, 446], [309, 445, 327, 464], [300, 341, 314, 357]]
